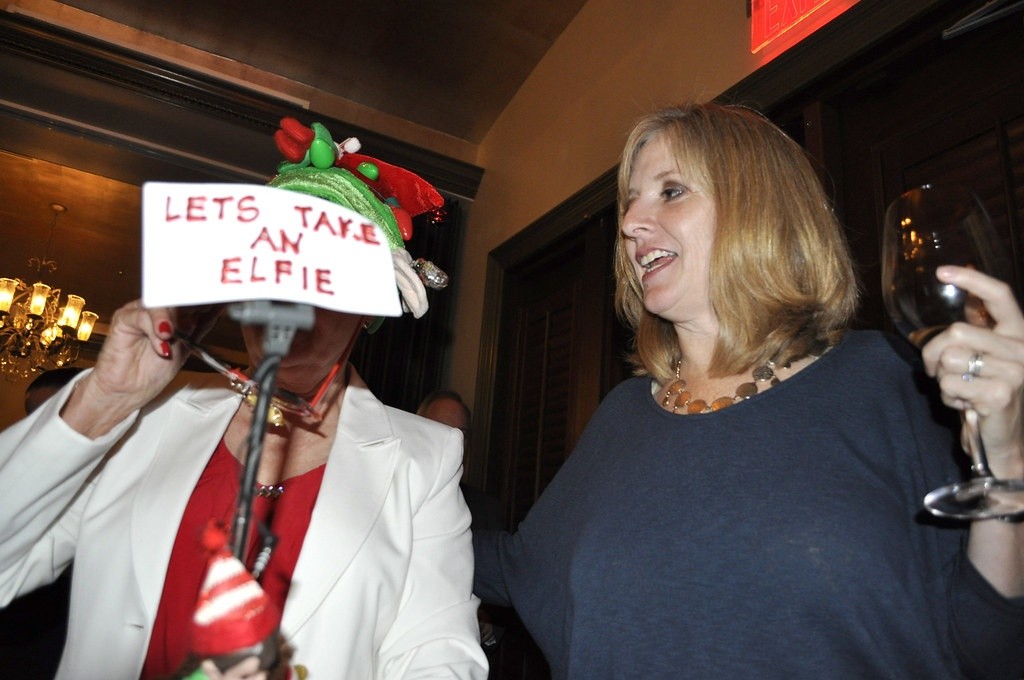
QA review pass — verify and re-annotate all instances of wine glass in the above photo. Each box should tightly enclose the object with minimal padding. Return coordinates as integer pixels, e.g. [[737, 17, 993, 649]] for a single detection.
[[881, 183, 1024, 520]]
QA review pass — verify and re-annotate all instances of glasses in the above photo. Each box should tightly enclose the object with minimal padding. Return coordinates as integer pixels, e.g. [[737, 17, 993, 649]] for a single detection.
[[181, 304, 369, 416]]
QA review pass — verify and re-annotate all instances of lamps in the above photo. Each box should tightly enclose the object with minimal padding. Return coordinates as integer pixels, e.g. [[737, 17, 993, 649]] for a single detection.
[[0, 203, 99, 382]]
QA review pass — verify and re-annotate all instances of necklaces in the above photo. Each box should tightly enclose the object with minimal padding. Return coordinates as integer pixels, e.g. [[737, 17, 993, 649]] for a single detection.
[[663, 350, 791, 413]]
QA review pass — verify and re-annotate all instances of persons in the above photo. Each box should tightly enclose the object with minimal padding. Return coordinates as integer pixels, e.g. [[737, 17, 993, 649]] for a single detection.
[[0, 367, 84, 680], [469, 97, 1024, 680], [0, 117, 489, 680], [415, 391, 505, 531]]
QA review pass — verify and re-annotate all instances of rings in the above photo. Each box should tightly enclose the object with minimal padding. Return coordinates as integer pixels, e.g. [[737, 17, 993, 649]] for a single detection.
[[961, 350, 983, 381]]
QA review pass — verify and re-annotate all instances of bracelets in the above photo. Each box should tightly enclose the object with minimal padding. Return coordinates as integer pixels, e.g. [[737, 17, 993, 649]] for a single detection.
[[993, 508, 1024, 524]]
